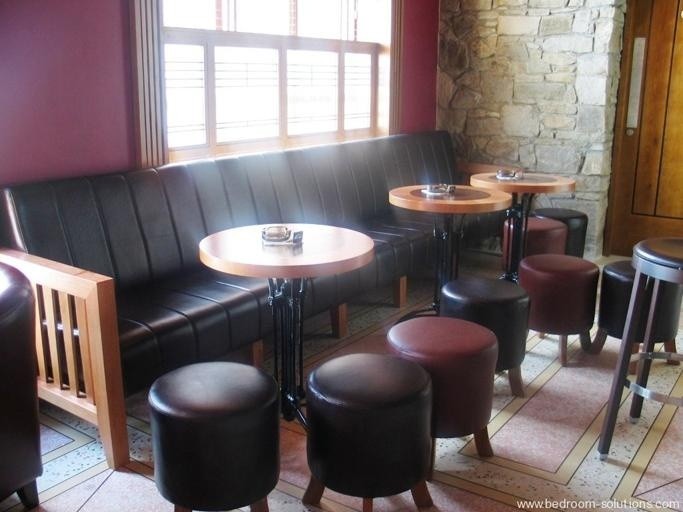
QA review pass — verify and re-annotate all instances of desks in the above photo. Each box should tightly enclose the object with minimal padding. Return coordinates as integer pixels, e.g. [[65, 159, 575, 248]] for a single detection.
[[386, 170, 577, 328]]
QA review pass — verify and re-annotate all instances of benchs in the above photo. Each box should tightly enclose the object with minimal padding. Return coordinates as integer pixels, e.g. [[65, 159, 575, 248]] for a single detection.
[[2, 126, 503, 463]]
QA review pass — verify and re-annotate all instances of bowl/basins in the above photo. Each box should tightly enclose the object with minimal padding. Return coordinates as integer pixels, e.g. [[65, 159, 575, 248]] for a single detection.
[[497, 169, 515, 178], [427, 183, 448, 193], [261, 226, 291, 242]]
